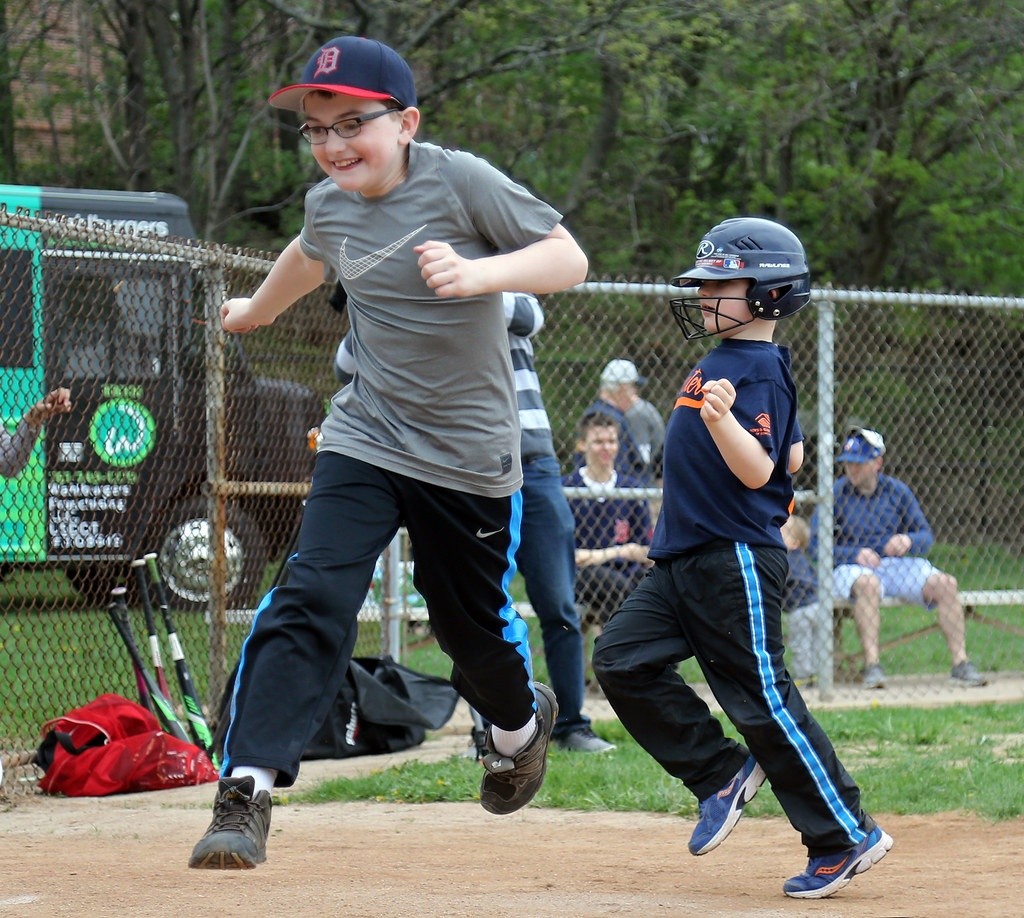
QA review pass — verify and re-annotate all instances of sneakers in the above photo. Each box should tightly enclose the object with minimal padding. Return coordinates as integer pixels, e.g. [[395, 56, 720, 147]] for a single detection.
[[478, 682, 559, 814], [557, 728, 615, 757], [784, 824, 893, 900], [189, 773, 272, 871], [466, 736, 482, 759], [950, 661, 988, 689], [863, 665, 885, 689], [687, 754, 765, 857]]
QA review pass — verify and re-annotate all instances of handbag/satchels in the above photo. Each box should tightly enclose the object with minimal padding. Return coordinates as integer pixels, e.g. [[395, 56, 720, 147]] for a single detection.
[[296, 658, 463, 761], [36, 693, 221, 797]]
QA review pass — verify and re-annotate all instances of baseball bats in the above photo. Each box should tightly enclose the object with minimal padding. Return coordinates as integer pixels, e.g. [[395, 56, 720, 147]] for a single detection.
[[109, 586, 156, 718], [145, 552, 222, 771], [105, 602, 194, 743], [130, 558, 172, 706]]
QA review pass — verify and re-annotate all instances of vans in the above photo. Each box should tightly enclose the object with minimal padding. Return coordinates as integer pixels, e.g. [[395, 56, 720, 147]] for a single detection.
[[0, 185, 327, 609]]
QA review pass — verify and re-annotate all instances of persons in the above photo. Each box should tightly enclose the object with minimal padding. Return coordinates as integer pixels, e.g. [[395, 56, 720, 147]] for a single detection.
[[780, 516, 818, 690], [561, 412, 654, 627], [575, 358, 665, 485], [592, 217, 896, 898], [809, 427, 989, 688], [187, 37, 587, 868], [0, 387, 71, 478], [336, 288, 618, 758]]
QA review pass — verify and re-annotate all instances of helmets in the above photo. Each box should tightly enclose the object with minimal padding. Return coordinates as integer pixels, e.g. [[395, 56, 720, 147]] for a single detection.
[[671, 218, 811, 319]]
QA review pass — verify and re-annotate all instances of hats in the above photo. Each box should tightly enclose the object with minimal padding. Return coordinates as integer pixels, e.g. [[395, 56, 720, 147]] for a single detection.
[[837, 434, 883, 465], [601, 358, 647, 388], [267, 36, 416, 109]]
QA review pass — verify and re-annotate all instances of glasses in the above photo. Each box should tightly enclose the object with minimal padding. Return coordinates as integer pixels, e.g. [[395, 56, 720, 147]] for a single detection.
[[298, 106, 406, 145]]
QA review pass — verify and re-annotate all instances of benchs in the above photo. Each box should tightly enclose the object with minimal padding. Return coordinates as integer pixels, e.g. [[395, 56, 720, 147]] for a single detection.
[[788, 587, 1024, 680], [205, 603, 542, 652]]
